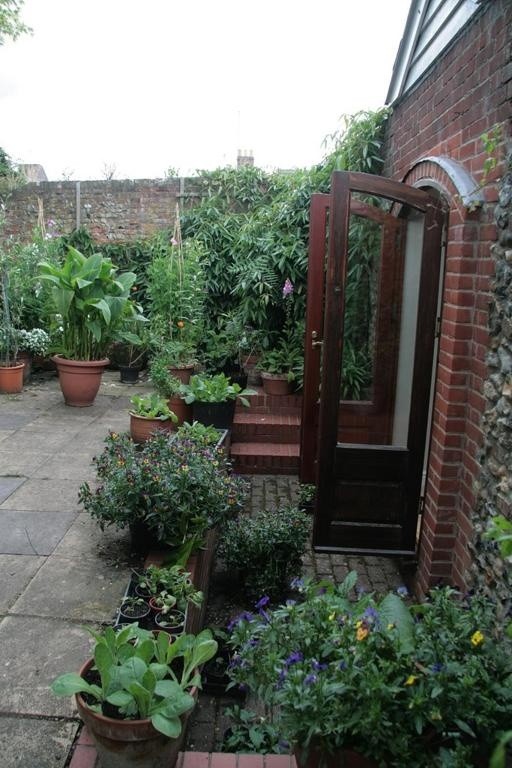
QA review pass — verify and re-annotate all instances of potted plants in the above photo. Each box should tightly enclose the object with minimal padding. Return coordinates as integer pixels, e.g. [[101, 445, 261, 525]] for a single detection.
[[148, 351, 239, 433], [119, 566, 205, 635], [114, 298, 147, 385], [151, 245, 296, 397], [40, 620, 219, 768], [33, 242, 148, 410], [0, 269, 24, 393], [128, 392, 181, 450]]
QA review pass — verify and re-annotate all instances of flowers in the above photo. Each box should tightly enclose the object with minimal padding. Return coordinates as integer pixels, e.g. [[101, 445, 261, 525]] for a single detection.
[[78, 422, 253, 547], [223, 569, 512, 768]]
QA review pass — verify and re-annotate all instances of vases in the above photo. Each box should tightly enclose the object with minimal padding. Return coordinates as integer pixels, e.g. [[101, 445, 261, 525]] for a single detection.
[[293, 744, 381, 767], [128, 512, 190, 555]]
